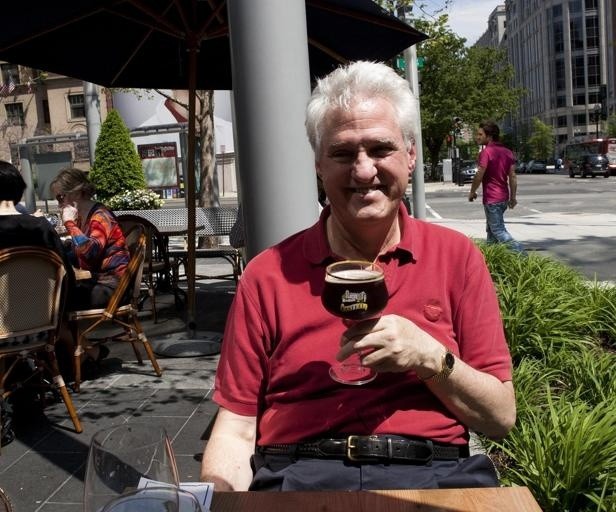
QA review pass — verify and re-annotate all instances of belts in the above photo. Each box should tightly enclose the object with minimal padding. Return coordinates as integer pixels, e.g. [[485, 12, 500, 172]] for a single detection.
[[258, 436, 470, 463]]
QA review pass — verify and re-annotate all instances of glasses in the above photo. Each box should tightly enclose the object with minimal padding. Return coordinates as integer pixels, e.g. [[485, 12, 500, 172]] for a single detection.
[[55, 194, 67, 204]]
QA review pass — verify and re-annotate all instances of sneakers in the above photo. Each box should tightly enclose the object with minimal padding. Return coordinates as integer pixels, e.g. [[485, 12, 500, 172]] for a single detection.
[[82, 345, 109, 371]]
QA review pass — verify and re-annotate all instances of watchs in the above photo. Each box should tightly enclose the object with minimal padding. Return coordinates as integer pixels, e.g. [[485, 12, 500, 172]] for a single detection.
[[418, 344, 456, 385]]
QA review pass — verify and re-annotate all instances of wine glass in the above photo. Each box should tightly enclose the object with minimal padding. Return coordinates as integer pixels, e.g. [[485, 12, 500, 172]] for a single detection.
[[320, 259, 389, 387]]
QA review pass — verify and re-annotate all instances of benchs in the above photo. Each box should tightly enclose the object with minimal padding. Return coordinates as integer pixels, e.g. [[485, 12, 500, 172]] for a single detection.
[[113, 207, 241, 286]]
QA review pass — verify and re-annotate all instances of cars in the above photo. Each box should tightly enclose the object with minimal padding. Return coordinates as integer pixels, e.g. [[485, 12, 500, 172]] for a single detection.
[[569, 155, 610, 178], [461, 160, 479, 182], [516, 159, 547, 174]]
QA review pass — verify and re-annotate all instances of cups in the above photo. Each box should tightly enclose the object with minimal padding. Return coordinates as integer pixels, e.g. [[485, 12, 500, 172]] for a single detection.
[[83, 421, 201, 512]]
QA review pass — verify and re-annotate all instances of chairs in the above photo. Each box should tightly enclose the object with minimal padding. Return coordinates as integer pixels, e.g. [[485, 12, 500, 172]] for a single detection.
[[0, 245, 82, 434], [66, 224, 163, 392], [114, 216, 179, 324], [0, 487, 13, 512]]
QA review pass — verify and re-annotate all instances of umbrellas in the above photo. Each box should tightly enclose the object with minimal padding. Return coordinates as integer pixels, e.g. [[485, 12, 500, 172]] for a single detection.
[[0, 1, 431, 322]]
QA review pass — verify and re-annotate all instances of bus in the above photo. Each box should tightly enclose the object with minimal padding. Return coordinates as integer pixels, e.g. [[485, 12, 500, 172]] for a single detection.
[[565, 138, 616, 172]]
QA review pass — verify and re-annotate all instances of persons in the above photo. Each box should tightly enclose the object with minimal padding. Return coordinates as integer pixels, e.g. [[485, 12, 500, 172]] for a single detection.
[[200, 61, 517, 492], [468, 121, 529, 258], [48, 167, 131, 378], [0, 159, 76, 449]]
